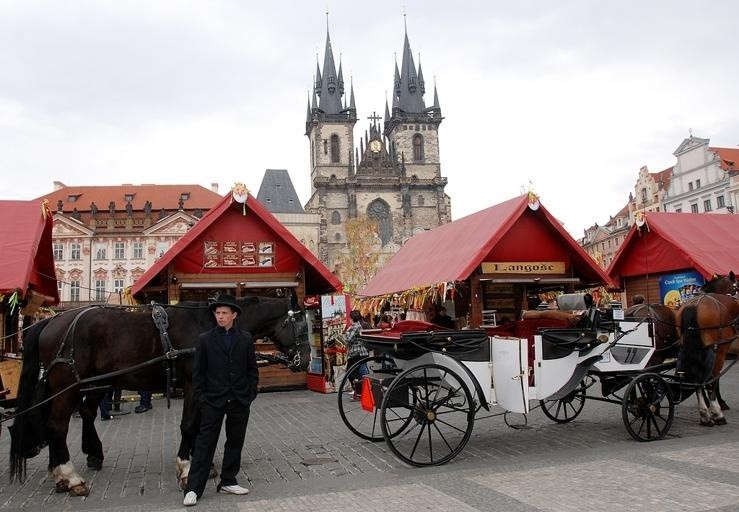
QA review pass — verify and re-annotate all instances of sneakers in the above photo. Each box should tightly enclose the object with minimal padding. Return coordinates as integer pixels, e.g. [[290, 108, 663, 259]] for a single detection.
[[348, 390, 355, 395], [220, 485, 249, 495], [183, 491, 197, 506]]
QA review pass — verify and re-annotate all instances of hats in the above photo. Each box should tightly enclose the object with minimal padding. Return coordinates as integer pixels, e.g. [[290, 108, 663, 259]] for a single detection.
[[209, 295, 242, 315]]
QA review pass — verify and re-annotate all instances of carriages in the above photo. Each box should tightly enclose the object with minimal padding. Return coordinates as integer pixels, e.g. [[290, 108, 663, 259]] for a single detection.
[[335, 268, 739, 467]]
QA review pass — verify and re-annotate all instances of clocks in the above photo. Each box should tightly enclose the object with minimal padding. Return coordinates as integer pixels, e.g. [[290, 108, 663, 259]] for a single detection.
[[370, 140, 382, 153]]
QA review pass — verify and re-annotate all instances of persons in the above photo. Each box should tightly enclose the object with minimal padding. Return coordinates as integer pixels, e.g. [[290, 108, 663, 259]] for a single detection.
[[182, 293, 258, 506], [340, 311, 368, 399], [134, 392, 153, 414], [632, 294, 645, 306], [362, 314, 406, 358], [430, 307, 454, 328], [499, 316, 510, 324]]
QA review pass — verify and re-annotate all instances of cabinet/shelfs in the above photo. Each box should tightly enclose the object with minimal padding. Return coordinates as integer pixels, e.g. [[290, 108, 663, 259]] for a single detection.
[[303, 294, 353, 392]]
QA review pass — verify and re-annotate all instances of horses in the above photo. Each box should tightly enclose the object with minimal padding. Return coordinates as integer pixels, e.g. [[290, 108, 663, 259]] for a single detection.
[[624, 270, 739, 417], [9, 288, 310, 496], [675, 292, 739, 427]]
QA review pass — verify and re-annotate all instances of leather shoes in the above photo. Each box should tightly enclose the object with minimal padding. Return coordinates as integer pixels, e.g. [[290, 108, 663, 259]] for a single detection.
[[135, 404, 152, 413]]
